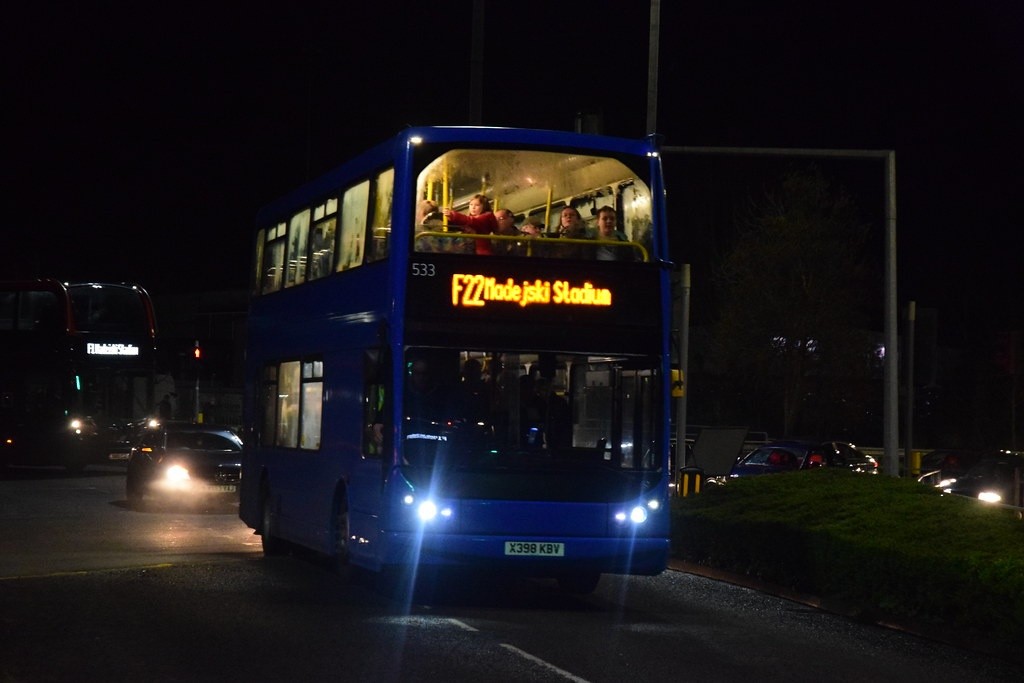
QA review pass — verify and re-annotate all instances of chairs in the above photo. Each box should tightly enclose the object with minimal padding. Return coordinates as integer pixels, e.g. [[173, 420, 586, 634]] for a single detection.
[[263, 249, 333, 293]]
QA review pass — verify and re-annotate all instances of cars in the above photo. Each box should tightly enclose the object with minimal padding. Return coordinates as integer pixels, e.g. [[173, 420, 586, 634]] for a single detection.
[[729, 437, 1024, 507], [125, 418, 244, 506]]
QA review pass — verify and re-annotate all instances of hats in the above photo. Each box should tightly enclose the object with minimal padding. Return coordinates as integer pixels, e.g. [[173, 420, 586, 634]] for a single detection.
[[529, 361, 556, 382]]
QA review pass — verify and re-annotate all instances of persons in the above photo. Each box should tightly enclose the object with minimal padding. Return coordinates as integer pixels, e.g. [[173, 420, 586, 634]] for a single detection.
[[369, 359, 571, 451], [417, 194, 634, 265]]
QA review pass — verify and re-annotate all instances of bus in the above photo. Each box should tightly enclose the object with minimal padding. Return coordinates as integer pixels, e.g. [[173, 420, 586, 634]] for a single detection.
[[239, 126, 689, 596], [0, 275, 160, 466]]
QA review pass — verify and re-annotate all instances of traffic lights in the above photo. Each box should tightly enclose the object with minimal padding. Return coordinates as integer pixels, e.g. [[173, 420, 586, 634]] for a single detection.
[[192, 346, 203, 360]]
